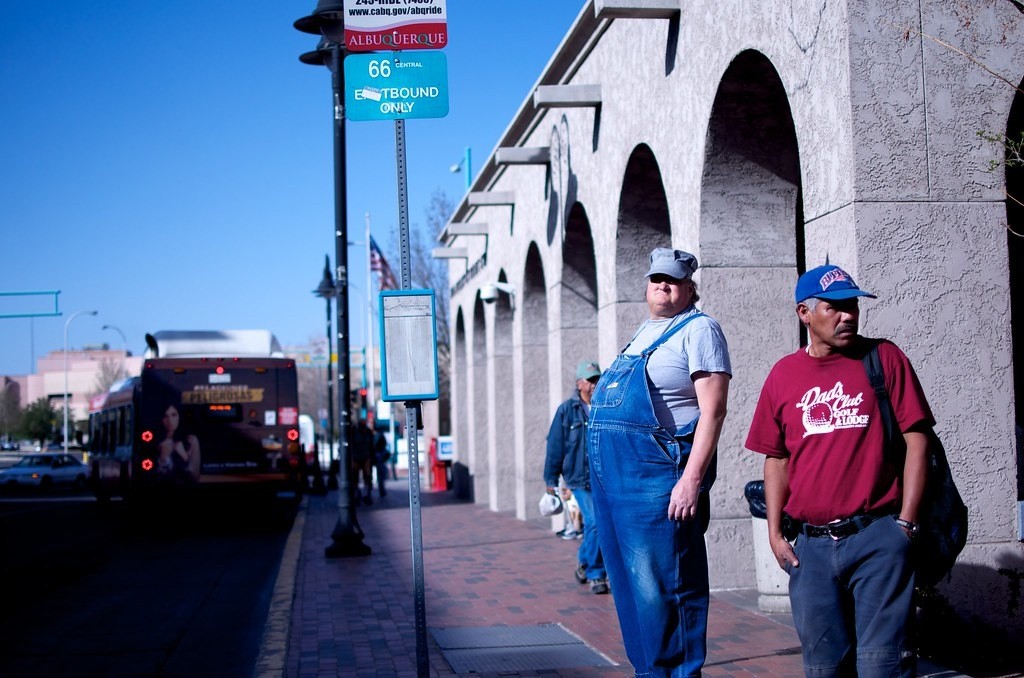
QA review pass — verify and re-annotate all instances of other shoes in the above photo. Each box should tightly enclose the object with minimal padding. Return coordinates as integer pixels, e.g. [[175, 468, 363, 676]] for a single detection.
[[555, 525, 585, 542], [363, 496, 373, 504], [590, 577, 608, 594], [575, 558, 588, 584]]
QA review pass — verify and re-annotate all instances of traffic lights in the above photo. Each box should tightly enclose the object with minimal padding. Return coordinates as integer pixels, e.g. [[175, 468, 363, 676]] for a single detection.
[[292, 0, 333, 70]]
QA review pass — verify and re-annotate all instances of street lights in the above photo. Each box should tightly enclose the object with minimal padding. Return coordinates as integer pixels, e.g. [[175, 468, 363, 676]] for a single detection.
[[313, 256, 338, 446]]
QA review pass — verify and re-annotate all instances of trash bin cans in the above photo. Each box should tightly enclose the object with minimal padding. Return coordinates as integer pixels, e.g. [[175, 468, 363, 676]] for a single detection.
[[744, 479, 798, 614]]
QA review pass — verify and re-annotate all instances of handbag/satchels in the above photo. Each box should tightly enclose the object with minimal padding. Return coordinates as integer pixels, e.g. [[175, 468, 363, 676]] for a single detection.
[[382, 451, 390, 462], [539, 491, 564, 518], [861, 335, 969, 589]]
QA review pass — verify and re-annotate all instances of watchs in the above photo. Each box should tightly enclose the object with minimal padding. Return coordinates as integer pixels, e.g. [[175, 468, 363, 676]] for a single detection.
[[896, 519, 919, 533]]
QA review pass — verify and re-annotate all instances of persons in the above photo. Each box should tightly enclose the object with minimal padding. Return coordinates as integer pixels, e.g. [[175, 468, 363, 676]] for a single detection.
[[155, 399, 201, 479], [744, 266, 937, 678], [352, 418, 391, 507], [588, 248, 732, 678], [543, 360, 609, 594]]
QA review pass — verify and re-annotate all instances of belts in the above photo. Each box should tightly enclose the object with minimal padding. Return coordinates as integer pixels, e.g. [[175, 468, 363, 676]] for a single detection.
[[793, 502, 898, 541]]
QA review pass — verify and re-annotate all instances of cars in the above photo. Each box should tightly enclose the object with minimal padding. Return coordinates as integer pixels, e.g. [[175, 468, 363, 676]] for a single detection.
[[0, 453, 90, 490]]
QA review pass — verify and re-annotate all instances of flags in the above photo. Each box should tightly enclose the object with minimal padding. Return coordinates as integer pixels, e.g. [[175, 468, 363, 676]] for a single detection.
[[370, 233, 401, 290]]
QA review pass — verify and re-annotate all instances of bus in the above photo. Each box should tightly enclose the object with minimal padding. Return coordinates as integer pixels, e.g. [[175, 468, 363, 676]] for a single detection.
[[90, 355, 301, 513]]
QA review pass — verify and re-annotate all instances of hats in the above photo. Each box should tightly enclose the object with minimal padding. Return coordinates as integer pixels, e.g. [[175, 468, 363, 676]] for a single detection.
[[576, 361, 602, 381], [796, 264, 877, 302], [644, 247, 698, 281]]
